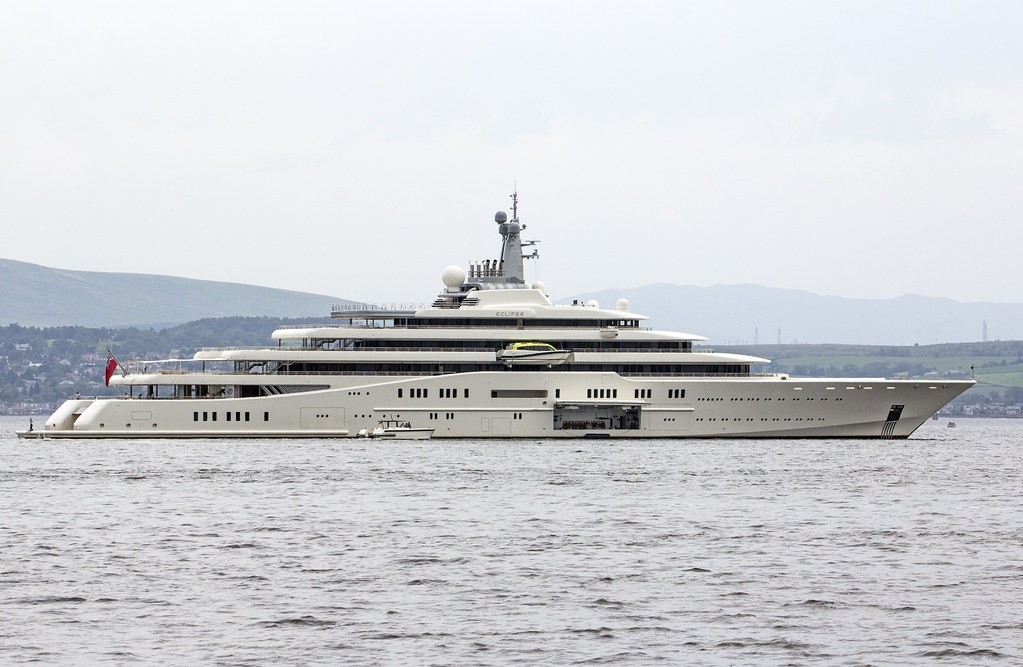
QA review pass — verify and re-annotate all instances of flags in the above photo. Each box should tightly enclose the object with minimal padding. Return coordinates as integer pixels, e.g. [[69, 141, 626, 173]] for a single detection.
[[105, 354, 118, 387]]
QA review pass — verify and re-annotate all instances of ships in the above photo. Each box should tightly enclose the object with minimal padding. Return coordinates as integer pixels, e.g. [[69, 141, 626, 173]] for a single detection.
[[15, 192, 976, 439]]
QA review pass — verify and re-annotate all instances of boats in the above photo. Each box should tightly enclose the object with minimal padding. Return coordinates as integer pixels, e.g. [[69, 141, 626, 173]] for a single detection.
[[372, 417, 435, 441], [947, 423, 956, 427], [496, 339, 571, 369]]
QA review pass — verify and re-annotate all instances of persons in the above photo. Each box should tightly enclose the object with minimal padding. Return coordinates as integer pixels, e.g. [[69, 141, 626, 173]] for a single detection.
[[29, 418, 33, 430], [95, 397, 100, 399], [75, 392, 80, 399], [138, 393, 154, 400], [125, 393, 128, 399]]
